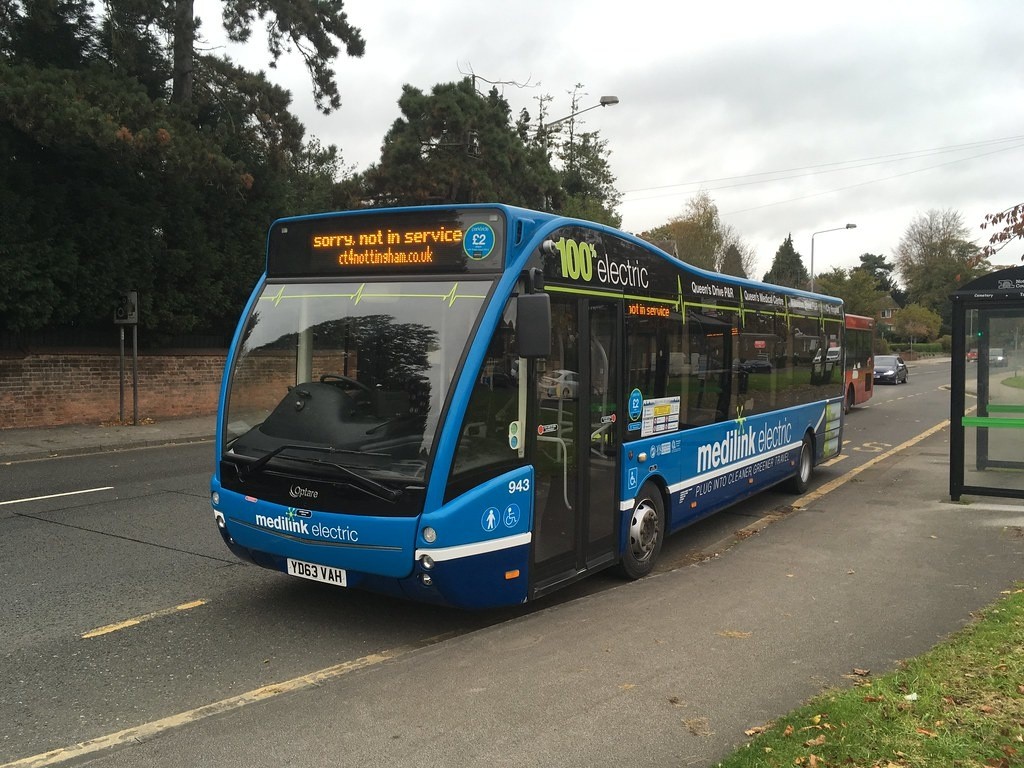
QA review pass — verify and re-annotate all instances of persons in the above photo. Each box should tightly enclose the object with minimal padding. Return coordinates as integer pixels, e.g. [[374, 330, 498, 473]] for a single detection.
[[737, 357, 752, 406]]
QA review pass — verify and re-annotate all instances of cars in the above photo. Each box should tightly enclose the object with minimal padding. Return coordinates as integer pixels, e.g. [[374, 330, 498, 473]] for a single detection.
[[733, 359, 773, 374], [770, 353, 801, 368], [873, 354, 909, 386], [967, 348, 979, 363], [538, 368, 594, 401], [989, 348, 1008, 367]]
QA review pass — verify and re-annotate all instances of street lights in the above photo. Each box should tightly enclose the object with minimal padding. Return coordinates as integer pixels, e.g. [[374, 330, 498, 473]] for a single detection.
[[810, 223, 858, 293], [543, 95, 620, 213]]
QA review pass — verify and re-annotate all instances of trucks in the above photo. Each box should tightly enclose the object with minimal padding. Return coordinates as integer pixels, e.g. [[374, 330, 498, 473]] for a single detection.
[[826, 346, 841, 363], [811, 348, 823, 365]]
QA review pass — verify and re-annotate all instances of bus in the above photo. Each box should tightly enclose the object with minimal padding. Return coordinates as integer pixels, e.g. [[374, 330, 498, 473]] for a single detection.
[[209, 201, 846, 610], [844, 311, 876, 413]]
